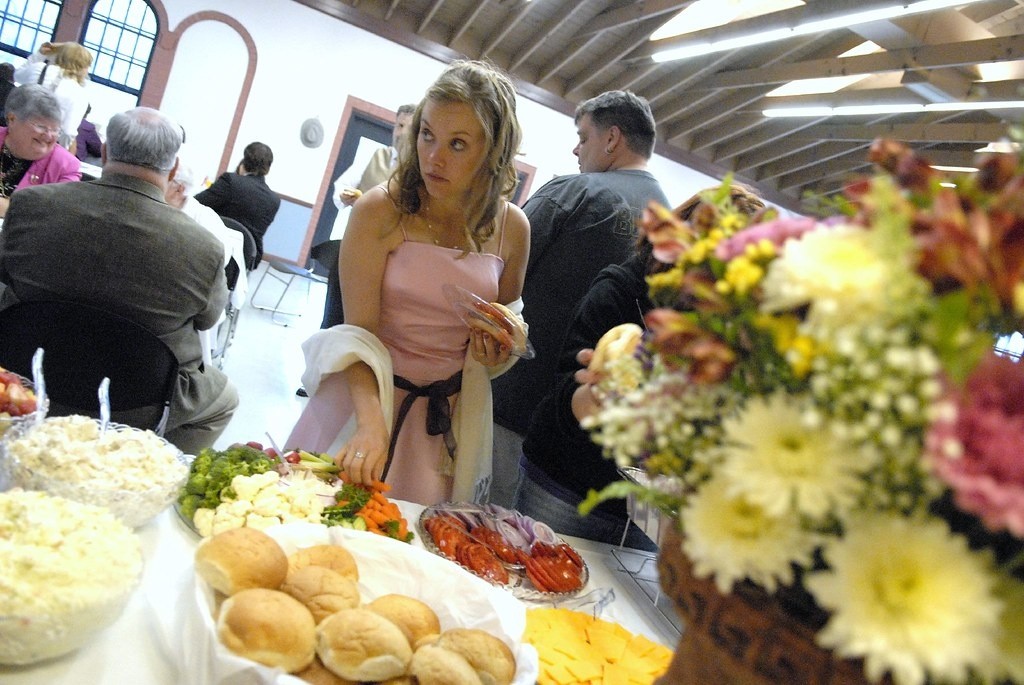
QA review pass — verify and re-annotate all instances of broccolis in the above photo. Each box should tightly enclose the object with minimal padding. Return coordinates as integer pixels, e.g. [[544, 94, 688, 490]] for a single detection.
[[193, 472, 324, 536], [179, 445, 280, 518]]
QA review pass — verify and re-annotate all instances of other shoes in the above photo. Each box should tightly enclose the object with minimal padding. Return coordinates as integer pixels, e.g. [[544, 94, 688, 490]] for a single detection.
[[296, 387, 308, 397]]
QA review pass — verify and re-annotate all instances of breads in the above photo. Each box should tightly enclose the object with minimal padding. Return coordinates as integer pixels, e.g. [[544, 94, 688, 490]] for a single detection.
[[192, 527, 517, 685]]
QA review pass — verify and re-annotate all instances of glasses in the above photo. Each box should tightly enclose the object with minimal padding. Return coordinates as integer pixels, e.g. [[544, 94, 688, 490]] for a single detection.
[[31, 121, 63, 137]]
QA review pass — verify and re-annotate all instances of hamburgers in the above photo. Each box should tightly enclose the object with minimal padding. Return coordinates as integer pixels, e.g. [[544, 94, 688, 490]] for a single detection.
[[463, 301, 527, 352]]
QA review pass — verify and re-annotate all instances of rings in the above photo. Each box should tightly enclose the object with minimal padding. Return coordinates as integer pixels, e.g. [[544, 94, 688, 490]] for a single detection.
[[355, 451, 366, 458], [477, 352, 486, 356]]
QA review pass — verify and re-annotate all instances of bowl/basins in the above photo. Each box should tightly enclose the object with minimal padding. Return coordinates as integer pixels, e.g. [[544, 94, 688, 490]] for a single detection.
[[272, 463, 343, 506], [1, 416, 190, 526], [0, 498, 142, 666], [0, 367, 50, 447]]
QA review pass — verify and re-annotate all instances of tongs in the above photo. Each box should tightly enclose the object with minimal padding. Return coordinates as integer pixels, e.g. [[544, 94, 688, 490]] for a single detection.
[[552, 587, 615, 621]]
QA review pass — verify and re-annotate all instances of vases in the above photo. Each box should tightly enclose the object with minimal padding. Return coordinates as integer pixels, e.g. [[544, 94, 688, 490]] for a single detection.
[[647, 520, 867, 685]]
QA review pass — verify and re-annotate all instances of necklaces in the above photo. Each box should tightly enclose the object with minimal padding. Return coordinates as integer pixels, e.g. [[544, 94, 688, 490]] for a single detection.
[[425, 201, 463, 251]]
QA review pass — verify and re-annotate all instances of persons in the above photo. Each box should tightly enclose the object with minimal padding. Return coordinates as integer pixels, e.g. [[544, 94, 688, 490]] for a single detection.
[[12, 40, 93, 143], [0, 83, 82, 220], [165, 151, 235, 366], [320, 104, 415, 329], [490, 90, 675, 512], [511, 184, 772, 554], [192, 140, 280, 272], [236, 160, 246, 174], [0, 107, 241, 453], [273, 60, 534, 511]]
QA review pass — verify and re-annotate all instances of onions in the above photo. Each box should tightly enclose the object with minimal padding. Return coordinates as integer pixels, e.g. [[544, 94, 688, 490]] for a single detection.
[[436, 499, 557, 552]]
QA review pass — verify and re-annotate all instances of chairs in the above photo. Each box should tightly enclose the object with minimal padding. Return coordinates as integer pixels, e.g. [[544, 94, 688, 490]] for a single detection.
[[0, 298, 180, 438], [250, 239, 341, 328]]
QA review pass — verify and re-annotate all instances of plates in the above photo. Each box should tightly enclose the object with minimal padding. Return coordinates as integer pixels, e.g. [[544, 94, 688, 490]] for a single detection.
[[442, 284, 536, 360], [420, 502, 589, 600], [173, 461, 411, 545]]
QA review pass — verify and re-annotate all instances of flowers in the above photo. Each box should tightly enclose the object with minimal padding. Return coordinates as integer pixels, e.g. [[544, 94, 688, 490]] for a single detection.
[[574, 120, 1024, 685]]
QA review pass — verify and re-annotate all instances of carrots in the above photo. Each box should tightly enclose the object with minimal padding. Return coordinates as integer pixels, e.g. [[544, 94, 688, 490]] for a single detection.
[[331, 471, 407, 542]]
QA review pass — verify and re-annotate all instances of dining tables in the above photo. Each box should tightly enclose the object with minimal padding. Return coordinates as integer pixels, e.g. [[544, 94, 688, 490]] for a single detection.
[[0, 455, 684, 685]]
